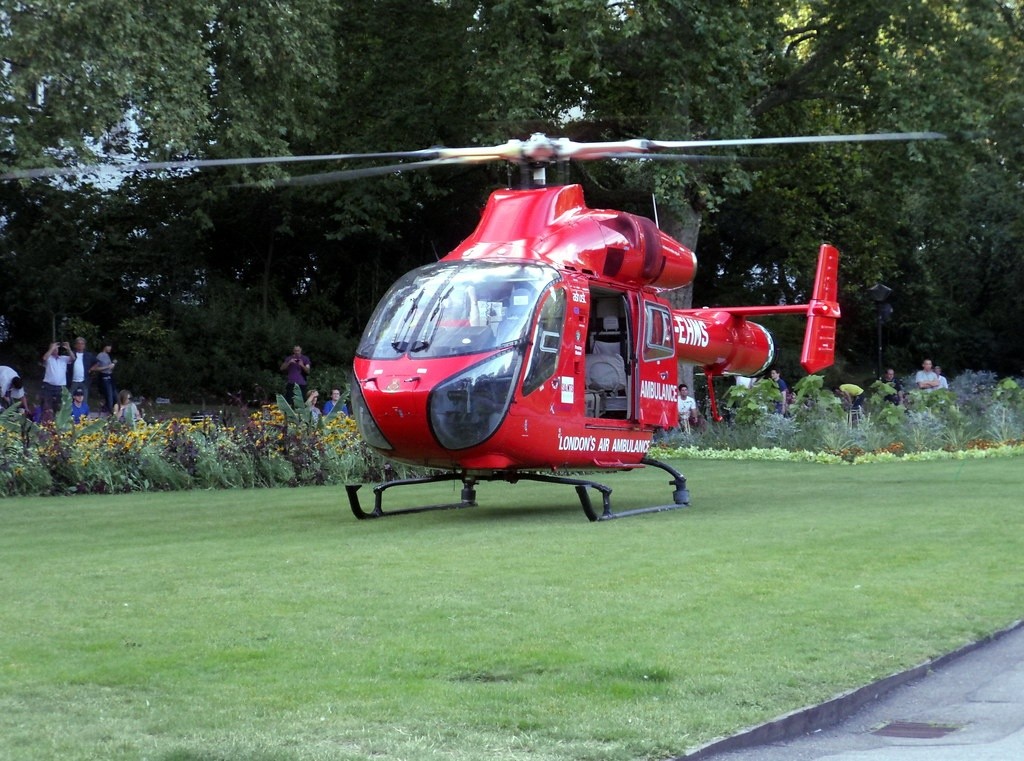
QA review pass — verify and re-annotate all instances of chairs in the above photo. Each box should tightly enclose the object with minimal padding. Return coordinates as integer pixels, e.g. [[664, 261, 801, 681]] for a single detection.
[[590, 311, 627, 412]]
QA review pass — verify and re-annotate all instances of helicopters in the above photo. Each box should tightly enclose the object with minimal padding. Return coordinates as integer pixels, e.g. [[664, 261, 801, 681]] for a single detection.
[[0, 128, 949, 520]]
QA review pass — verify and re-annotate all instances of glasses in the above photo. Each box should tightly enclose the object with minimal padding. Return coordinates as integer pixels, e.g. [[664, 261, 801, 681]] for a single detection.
[[128, 398, 131, 400]]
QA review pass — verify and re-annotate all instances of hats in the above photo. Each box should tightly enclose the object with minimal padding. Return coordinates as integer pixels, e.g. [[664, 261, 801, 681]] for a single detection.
[[73, 388, 84, 396]]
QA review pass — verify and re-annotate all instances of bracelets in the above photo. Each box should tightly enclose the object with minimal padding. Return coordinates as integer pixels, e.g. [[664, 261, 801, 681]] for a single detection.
[[471, 302, 477, 305]]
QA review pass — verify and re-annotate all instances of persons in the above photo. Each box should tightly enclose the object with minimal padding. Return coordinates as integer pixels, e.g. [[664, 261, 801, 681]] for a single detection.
[[916, 360, 938, 392], [114, 390, 141, 425], [934, 366, 947, 389], [678, 384, 697, 425], [67, 337, 98, 402], [323, 389, 348, 417], [70, 389, 89, 424], [41, 341, 75, 415], [881, 369, 903, 406], [94, 341, 119, 413], [281, 343, 311, 409], [833, 384, 866, 419], [305, 390, 321, 415], [771, 368, 787, 417], [435, 286, 480, 327], [0, 366, 30, 414]]
